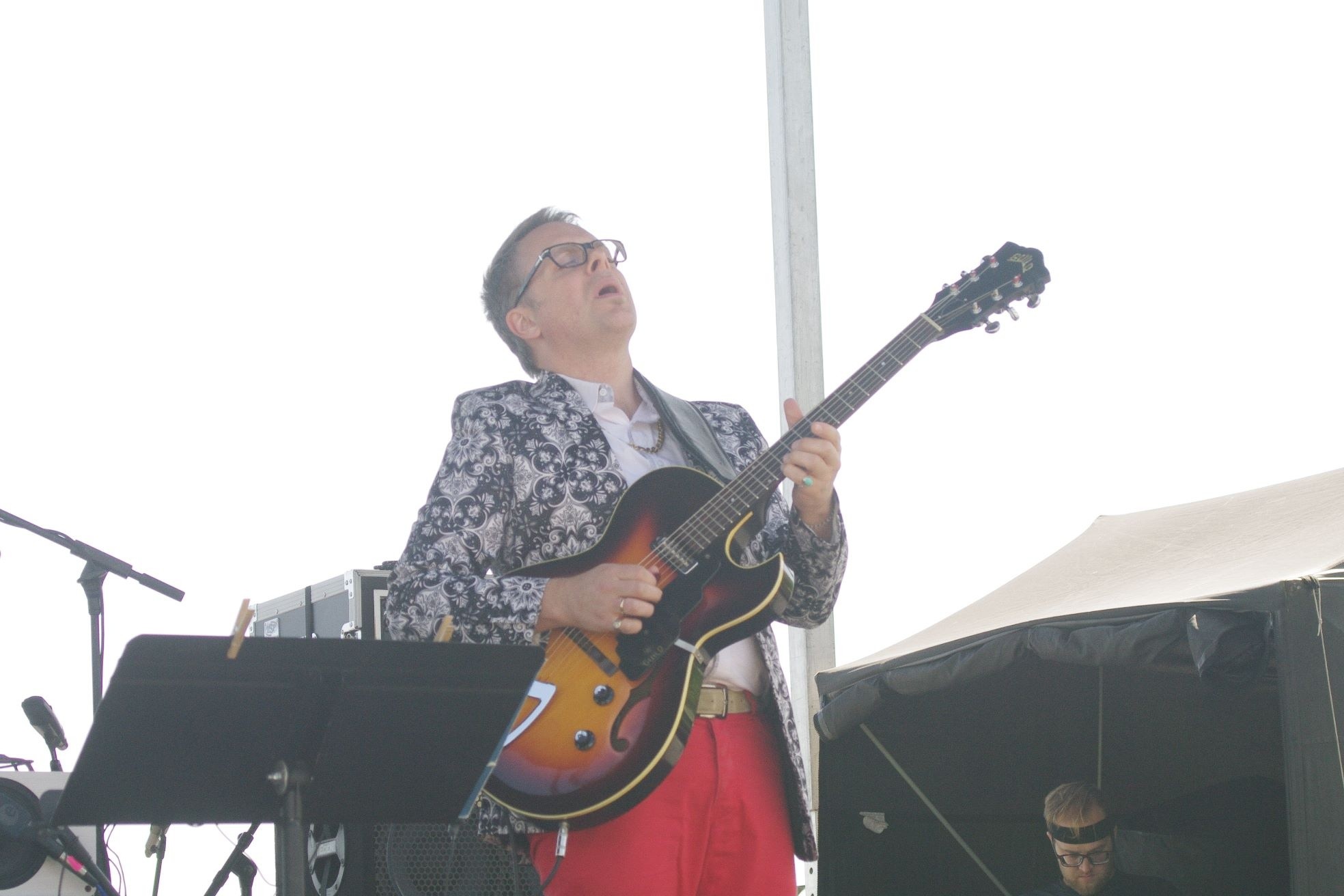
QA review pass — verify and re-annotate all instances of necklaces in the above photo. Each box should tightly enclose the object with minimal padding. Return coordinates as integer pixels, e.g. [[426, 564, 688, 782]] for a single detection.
[[628, 418, 665, 453]]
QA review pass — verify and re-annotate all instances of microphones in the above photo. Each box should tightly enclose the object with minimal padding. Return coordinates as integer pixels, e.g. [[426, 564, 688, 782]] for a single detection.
[[20, 696, 69, 750], [145, 823, 170, 858], [0, 803, 98, 886]]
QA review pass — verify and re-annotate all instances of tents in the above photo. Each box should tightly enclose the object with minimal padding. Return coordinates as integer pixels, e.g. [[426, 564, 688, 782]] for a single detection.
[[816, 478, 1344, 896]]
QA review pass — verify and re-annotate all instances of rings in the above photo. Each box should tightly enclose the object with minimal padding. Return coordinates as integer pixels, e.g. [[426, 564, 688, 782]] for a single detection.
[[620, 599, 625, 615], [796, 475, 813, 489], [614, 616, 625, 633]]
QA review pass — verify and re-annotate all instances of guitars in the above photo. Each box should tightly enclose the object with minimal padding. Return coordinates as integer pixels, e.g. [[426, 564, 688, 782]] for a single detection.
[[429, 240, 1050, 828]]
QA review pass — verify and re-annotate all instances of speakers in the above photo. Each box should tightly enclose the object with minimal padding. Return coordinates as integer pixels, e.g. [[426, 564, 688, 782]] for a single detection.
[[0, 770, 107, 896], [274, 821, 546, 896]]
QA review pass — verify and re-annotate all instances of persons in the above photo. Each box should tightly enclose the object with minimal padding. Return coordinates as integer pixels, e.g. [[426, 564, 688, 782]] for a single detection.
[[383, 207, 852, 896], [1028, 779, 1179, 896]]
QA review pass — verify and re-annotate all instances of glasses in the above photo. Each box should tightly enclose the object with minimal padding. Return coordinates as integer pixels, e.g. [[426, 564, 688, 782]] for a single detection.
[[513, 239, 627, 309], [1051, 840, 1115, 867]]
